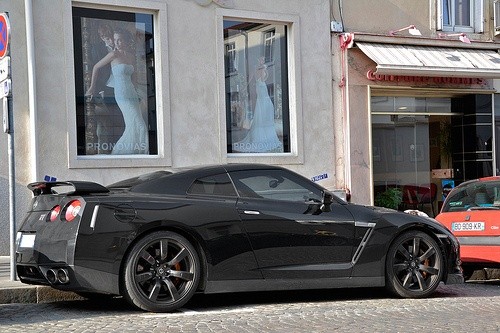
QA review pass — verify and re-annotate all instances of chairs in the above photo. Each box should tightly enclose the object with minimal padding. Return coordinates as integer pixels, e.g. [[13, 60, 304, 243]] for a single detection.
[[374, 183, 436, 219]]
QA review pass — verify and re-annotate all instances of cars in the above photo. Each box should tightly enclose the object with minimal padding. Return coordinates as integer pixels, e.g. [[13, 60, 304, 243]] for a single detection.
[[434, 176, 500, 280]]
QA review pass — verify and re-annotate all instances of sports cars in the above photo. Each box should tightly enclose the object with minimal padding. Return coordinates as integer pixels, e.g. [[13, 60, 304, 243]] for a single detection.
[[14, 164, 463, 314]]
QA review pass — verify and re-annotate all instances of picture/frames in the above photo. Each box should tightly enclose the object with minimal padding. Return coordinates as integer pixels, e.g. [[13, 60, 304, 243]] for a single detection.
[[409, 144, 425, 162]]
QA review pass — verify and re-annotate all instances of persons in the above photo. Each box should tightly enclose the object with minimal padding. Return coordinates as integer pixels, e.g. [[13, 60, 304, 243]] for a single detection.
[[85, 23, 149, 154], [232, 53, 284, 152]]
